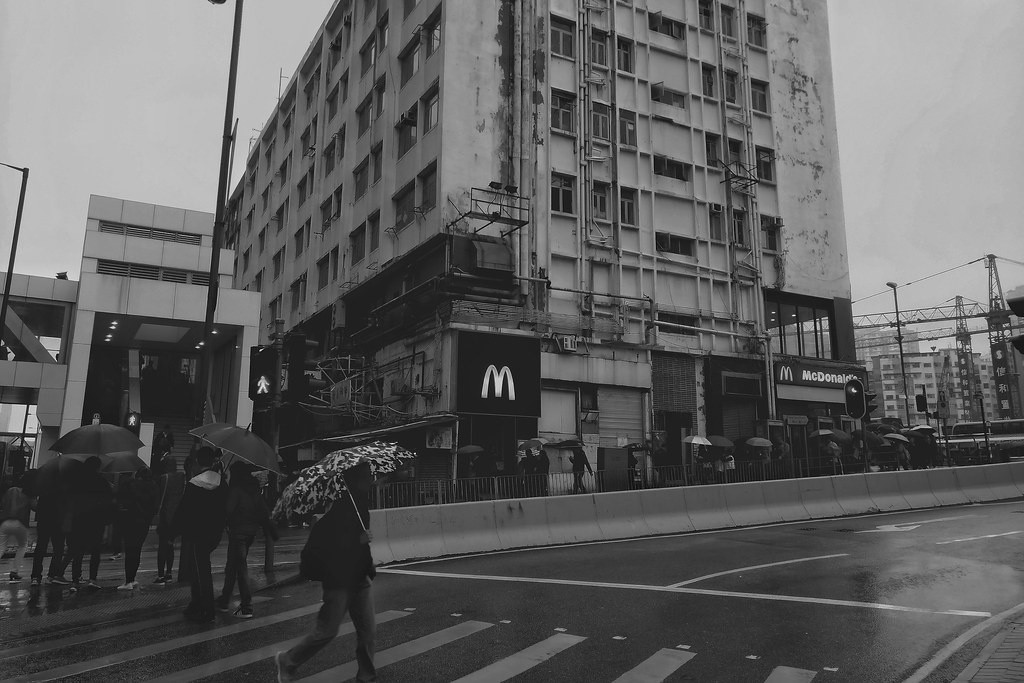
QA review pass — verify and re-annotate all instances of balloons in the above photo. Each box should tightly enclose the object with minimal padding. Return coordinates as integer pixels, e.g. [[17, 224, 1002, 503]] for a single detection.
[[518, 436, 549, 457]]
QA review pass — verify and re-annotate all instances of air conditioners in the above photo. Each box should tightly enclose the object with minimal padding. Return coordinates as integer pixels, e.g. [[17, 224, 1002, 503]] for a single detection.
[[330, 39, 339, 49], [344, 15, 351, 24], [709, 203, 722, 213], [769, 216, 783, 226], [400, 111, 413, 123]]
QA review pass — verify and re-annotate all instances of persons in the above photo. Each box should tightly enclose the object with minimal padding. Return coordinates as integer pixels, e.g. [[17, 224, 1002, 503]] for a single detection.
[[466, 451, 485, 499], [693, 435, 935, 485], [518, 450, 550, 495], [275, 460, 379, 683], [155, 425, 176, 455], [0, 456, 187, 592], [629, 454, 639, 486], [154, 455, 297, 624], [568, 446, 595, 494]]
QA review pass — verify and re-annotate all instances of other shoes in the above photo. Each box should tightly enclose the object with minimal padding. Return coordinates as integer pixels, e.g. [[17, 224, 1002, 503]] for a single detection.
[[45, 577, 53, 584], [117, 581, 137, 591], [165, 576, 173, 583], [87, 581, 101, 589], [152, 577, 165, 586], [9, 572, 22, 584], [52, 576, 69, 585], [70, 587, 78, 591], [30, 577, 38, 586]]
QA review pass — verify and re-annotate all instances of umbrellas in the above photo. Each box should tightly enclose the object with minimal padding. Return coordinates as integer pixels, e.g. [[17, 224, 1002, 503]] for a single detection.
[[268, 441, 420, 531], [30, 421, 149, 490], [447, 443, 485, 461], [189, 420, 298, 494], [679, 421, 936, 452], [553, 440, 588, 457], [621, 443, 651, 454]]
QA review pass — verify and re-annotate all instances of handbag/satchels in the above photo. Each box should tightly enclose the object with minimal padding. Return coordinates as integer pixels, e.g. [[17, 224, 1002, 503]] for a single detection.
[[142, 474, 175, 522], [702, 461, 712, 468]]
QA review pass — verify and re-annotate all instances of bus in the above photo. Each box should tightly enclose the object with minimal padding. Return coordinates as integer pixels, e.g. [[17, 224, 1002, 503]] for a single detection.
[[952, 419, 1024, 458]]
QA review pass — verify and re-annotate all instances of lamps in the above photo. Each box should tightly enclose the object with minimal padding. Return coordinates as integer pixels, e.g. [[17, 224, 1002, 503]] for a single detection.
[[488, 181, 502, 191], [505, 185, 519, 194]]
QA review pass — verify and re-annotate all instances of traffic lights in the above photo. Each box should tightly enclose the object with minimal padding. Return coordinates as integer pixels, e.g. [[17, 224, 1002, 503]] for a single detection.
[[248, 345, 277, 402], [862, 392, 879, 424], [843, 379, 866, 419]]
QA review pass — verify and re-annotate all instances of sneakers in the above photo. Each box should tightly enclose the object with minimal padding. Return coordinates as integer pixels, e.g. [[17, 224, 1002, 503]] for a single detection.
[[233, 607, 252, 619], [275, 649, 290, 683], [214, 599, 229, 613]]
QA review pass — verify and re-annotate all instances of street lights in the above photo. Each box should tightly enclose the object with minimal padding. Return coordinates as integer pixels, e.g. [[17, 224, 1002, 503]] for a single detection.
[[887, 282, 911, 429], [195, 0, 247, 449]]
